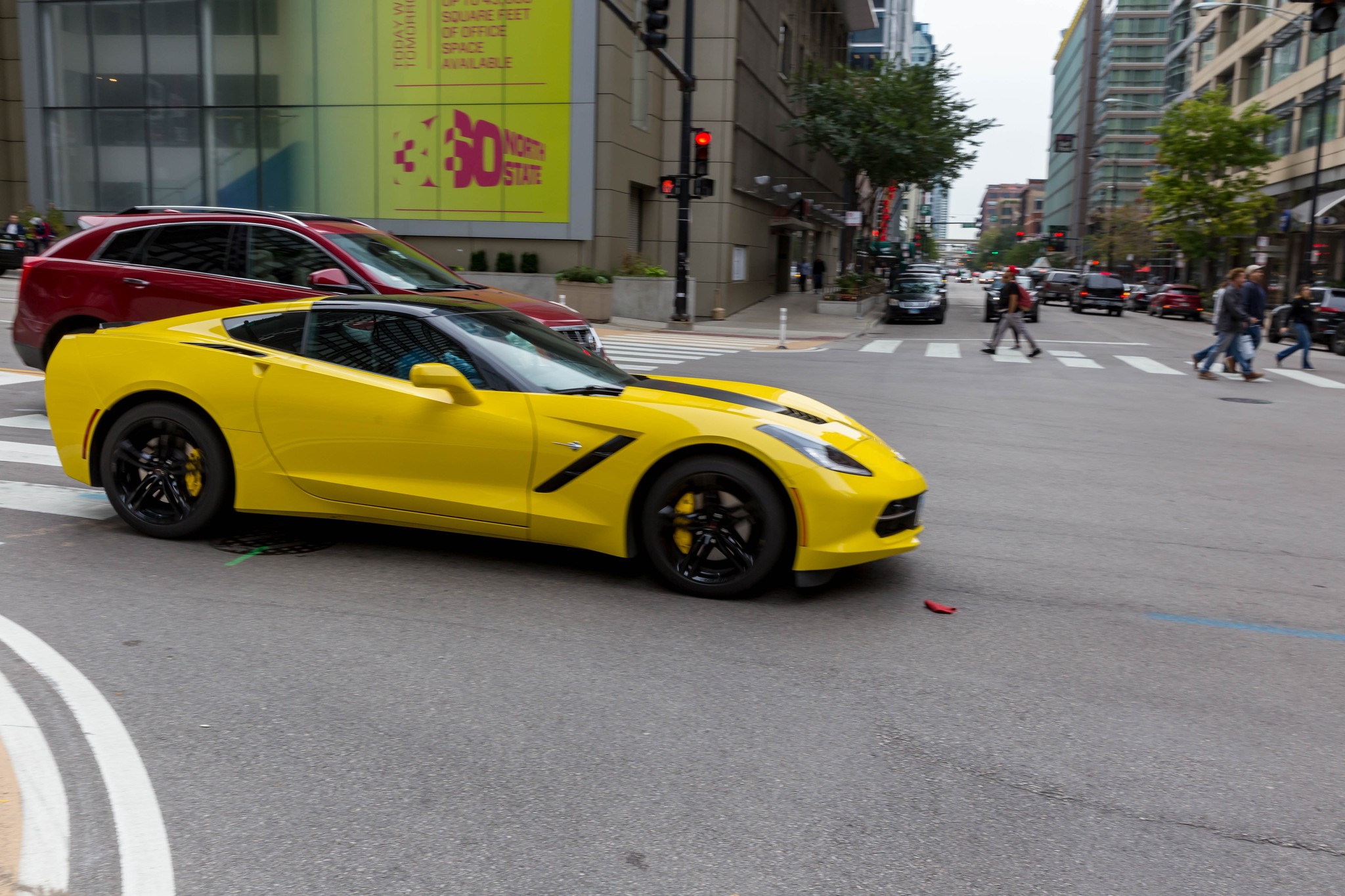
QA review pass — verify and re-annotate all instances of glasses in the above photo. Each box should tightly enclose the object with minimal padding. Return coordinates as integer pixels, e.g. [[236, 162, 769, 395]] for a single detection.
[[1002, 276, 1007, 279]]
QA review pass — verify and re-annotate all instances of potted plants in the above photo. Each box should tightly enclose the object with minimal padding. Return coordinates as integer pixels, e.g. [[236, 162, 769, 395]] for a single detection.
[[835, 271, 860, 294], [553, 263, 614, 323], [614, 251, 697, 322]]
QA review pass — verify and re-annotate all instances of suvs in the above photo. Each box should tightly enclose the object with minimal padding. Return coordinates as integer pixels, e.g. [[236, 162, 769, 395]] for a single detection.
[[1268, 285, 1345, 351], [1037, 272, 1078, 305], [1069, 273, 1126, 316], [9, 206, 612, 367], [1148, 283, 1203, 320]]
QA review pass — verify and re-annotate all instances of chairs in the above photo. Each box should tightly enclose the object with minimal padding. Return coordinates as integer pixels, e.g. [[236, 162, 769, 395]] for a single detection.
[[250, 249, 279, 283], [292, 249, 314, 287]]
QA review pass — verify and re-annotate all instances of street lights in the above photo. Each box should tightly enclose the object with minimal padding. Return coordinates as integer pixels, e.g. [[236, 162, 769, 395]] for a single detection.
[[1089, 154, 1118, 271], [1193, 2, 1333, 284]]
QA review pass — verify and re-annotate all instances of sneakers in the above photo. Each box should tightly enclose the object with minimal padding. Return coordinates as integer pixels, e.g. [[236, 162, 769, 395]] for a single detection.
[[1009, 346, 1022, 350], [983, 342, 992, 348]]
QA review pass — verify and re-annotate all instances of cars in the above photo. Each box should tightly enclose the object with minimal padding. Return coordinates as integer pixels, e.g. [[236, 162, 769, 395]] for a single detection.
[[982, 277, 1044, 322], [1122, 283, 1160, 311], [957, 271, 972, 283], [974, 271, 1001, 283], [884, 263, 948, 324], [1003, 257, 1055, 284], [790, 266, 800, 282]]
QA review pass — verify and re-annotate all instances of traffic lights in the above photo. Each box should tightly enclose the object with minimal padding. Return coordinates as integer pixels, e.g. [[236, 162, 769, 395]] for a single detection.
[[643, 0, 670, 49], [693, 178, 716, 196], [694, 131, 710, 176], [657, 176, 680, 196], [1017, 231, 1023, 240]]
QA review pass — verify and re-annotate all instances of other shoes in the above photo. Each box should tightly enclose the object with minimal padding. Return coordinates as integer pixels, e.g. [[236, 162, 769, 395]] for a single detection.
[[1191, 354, 1199, 371], [1197, 371, 1219, 380], [1303, 367, 1317, 371], [1275, 354, 1282, 367], [980, 349, 995, 354], [1026, 348, 1041, 357], [1220, 356, 1241, 373], [1242, 372, 1264, 381]]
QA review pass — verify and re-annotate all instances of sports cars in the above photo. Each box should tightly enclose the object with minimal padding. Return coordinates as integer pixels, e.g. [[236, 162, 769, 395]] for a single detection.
[[43, 291, 929, 601]]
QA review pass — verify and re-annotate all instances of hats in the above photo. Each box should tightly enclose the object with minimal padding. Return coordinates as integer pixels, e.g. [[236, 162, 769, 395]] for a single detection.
[[1245, 265, 1267, 276]]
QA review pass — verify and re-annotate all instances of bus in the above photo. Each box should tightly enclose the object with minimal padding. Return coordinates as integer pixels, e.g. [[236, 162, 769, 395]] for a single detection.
[[944, 260, 959, 275], [853, 240, 915, 275]]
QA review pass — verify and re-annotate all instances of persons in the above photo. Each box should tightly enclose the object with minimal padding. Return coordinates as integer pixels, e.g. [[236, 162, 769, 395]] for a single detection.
[[889, 263, 901, 290], [798, 256, 811, 292], [979, 270, 1041, 357], [812, 253, 827, 294], [1191, 264, 1268, 382], [0, 214, 51, 256], [1274, 283, 1321, 371]]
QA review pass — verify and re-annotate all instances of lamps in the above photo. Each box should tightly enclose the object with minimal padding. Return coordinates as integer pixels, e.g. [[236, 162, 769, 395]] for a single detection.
[[756, 174, 848, 221]]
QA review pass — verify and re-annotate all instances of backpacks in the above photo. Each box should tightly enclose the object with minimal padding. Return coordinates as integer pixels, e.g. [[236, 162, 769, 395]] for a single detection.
[[1007, 280, 1032, 311]]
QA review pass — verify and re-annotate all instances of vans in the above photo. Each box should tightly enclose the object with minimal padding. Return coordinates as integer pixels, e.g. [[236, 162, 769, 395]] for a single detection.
[[1083, 260, 1109, 273]]
